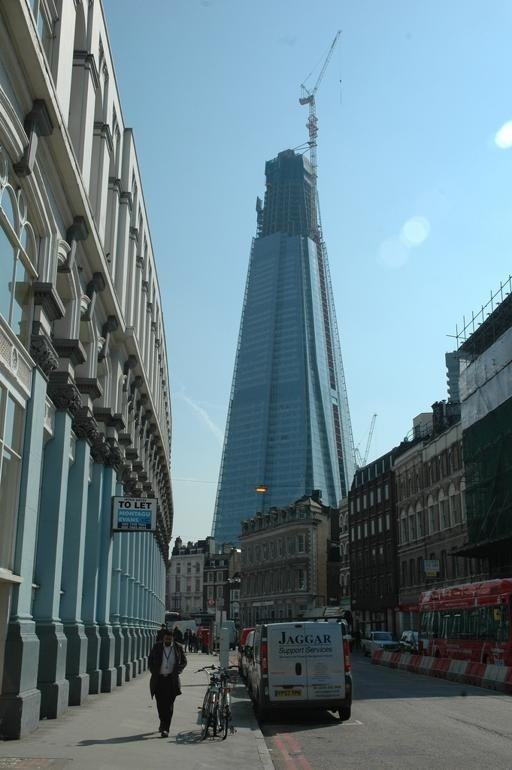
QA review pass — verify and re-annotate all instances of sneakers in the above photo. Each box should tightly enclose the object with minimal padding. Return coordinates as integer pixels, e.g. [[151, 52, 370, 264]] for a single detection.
[[157, 724, 168, 737]]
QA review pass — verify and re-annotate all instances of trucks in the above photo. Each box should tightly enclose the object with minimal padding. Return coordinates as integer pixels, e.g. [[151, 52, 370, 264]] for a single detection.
[[212, 621, 237, 652]]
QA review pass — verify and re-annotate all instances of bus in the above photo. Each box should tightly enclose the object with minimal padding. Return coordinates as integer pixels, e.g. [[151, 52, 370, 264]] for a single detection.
[[416, 578, 512, 666]]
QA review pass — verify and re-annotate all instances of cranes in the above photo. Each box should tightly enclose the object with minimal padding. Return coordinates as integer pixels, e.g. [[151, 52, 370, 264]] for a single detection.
[[299, 28, 346, 240], [351, 415, 379, 470]]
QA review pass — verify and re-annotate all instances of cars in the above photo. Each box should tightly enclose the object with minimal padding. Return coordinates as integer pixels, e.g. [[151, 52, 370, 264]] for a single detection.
[[360, 631, 402, 654], [401, 630, 420, 654]]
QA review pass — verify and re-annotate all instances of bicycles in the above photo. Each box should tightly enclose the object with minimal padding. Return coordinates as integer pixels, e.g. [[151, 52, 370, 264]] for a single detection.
[[195, 663, 239, 742]]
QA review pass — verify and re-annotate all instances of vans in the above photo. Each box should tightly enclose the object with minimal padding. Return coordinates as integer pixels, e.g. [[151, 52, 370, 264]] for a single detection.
[[239, 618, 352, 721]]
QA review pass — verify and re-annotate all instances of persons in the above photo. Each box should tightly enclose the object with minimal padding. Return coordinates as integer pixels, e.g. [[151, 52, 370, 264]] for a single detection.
[[148, 628, 187, 738], [156, 621, 203, 654]]
